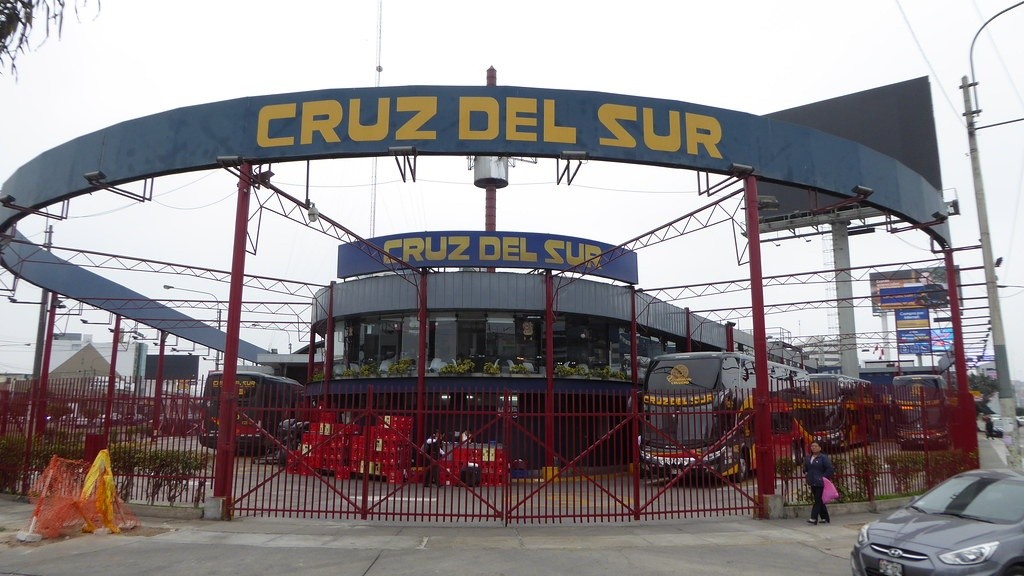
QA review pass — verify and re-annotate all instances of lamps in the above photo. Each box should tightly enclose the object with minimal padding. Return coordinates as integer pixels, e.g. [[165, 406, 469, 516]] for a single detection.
[[203, 351, 223, 360], [308, 202, 319, 222], [108, 320, 139, 333], [217, 155, 272, 190], [933, 238, 982, 253], [80, 311, 113, 325], [0, 193, 70, 220], [188, 347, 210, 356], [83, 170, 155, 203], [886, 210, 948, 234], [953, 255, 1004, 270], [956, 274, 999, 370], [697, 162, 755, 196], [153, 335, 178, 346], [387, 145, 419, 183], [132, 329, 160, 340], [808, 185, 874, 215], [556, 150, 589, 187], [10, 290, 48, 305], [171, 342, 195, 352], [216, 362, 224, 366]]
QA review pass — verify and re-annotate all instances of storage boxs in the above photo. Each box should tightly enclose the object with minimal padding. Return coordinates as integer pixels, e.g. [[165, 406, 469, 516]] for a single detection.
[[511, 459, 529, 470], [282, 400, 512, 487]]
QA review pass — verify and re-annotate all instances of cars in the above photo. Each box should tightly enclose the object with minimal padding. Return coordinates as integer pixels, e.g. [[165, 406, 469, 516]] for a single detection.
[[58, 414, 147, 427], [1015, 416, 1024, 426], [992, 416, 1004, 438], [849, 467, 1024, 576]]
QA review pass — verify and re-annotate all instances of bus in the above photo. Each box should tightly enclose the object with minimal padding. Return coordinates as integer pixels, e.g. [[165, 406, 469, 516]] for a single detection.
[[806, 373, 878, 454], [199, 370, 305, 455], [891, 374, 952, 452], [637, 352, 811, 483]]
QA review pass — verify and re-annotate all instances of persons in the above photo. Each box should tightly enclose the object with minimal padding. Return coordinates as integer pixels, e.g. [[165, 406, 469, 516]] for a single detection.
[[985, 418, 994, 438], [803, 441, 833, 525], [424, 431, 446, 488], [462, 426, 473, 444]]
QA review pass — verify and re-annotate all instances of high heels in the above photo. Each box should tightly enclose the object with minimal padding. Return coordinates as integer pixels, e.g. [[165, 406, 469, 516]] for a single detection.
[[806, 518, 817, 525], [819, 518, 830, 523]]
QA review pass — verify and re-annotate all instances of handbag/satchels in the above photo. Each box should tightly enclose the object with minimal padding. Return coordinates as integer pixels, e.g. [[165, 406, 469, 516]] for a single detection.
[[822, 477, 839, 504]]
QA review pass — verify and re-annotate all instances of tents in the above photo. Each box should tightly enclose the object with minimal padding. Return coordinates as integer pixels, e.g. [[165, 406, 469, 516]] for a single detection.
[[48, 344, 121, 380]]
